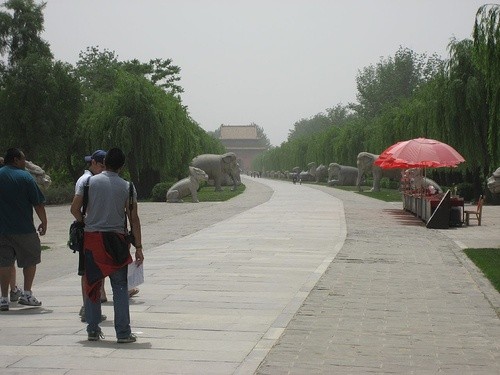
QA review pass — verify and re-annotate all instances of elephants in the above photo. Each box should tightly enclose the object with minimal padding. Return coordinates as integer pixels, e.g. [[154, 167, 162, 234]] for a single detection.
[[192, 152, 237, 191], [326, 163, 358, 185], [355, 152, 403, 193]]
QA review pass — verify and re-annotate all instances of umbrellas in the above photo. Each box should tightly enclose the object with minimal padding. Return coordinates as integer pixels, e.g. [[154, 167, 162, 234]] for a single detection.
[[375, 137, 466, 196]]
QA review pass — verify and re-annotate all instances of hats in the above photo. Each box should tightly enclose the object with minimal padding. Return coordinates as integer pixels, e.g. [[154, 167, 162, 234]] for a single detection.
[[85, 150, 107, 164]]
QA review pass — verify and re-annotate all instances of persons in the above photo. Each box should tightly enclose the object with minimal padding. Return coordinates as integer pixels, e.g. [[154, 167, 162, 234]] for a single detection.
[[0, 147, 47, 310], [70, 147, 145, 343], [74, 150, 140, 323], [250, 168, 262, 179]]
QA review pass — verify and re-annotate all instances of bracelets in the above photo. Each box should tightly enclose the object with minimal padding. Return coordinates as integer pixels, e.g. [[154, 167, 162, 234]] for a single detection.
[[136, 245, 142, 249]]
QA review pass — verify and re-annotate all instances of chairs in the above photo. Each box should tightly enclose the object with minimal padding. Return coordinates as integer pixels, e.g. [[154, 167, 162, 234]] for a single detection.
[[463, 194, 485, 226]]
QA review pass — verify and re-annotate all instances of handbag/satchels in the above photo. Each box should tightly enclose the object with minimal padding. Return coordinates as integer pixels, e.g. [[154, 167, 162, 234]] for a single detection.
[[129, 231, 138, 249], [68, 221, 84, 253]]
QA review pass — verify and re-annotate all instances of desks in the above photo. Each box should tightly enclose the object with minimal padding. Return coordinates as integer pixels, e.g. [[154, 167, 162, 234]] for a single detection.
[[404, 191, 464, 226]]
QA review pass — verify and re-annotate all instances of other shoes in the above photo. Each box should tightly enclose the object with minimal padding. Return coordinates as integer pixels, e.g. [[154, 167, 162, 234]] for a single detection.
[[0, 300, 9, 310], [117, 333, 137, 342], [79, 306, 106, 322], [10, 288, 23, 300], [87, 325, 105, 340], [128, 288, 138, 297], [18, 294, 42, 305], [100, 298, 107, 303]]
